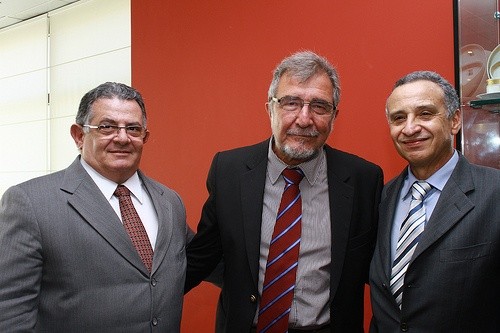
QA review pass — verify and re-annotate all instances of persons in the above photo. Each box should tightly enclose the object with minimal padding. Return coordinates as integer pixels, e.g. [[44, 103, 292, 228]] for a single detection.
[[0, 81, 228, 333], [183, 51, 386, 333], [366, 70, 500, 333]]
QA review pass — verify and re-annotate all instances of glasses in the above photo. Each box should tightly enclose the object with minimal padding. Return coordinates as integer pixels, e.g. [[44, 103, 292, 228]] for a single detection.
[[272, 95, 335, 115], [84, 123, 146, 137]]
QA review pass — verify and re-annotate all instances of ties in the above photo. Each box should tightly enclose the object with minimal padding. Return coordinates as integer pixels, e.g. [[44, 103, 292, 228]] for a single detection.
[[256, 167, 304, 333], [114, 185, 154, 273], [390, 182, 432, 311]]
